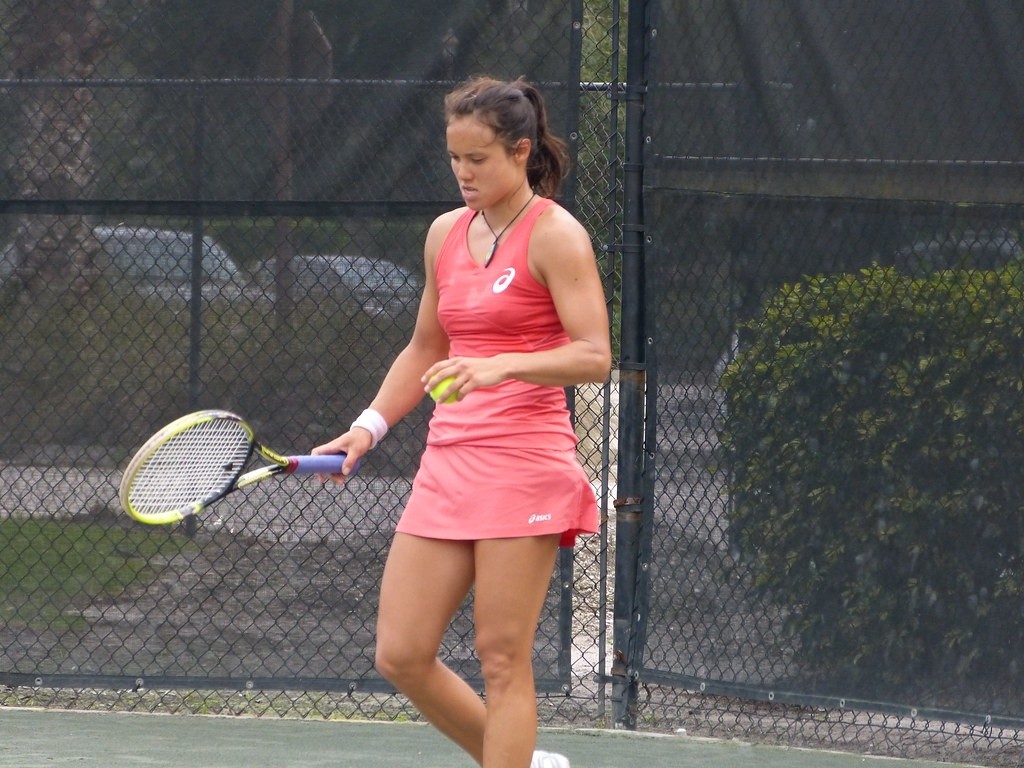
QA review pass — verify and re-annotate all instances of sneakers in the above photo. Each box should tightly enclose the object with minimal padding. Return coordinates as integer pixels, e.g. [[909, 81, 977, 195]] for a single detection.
[[529, 750, 569, 768]]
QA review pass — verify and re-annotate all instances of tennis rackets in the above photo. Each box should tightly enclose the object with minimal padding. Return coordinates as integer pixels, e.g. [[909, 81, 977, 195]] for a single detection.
[[119, 407, 364, 526]]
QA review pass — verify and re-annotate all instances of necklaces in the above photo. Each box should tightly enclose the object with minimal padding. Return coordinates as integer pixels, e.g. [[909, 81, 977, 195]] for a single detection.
[[481, 191, 536, 268]]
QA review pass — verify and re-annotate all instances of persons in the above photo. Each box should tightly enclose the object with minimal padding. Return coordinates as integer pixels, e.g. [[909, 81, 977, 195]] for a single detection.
[[312, 77, 611, 768]]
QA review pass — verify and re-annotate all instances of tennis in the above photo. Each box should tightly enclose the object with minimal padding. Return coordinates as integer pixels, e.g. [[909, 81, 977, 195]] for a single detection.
[[428, 368, 460, 404]]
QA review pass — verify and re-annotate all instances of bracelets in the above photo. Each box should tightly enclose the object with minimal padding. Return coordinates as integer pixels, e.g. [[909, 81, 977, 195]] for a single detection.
[[349, 408, 388, 451]]
